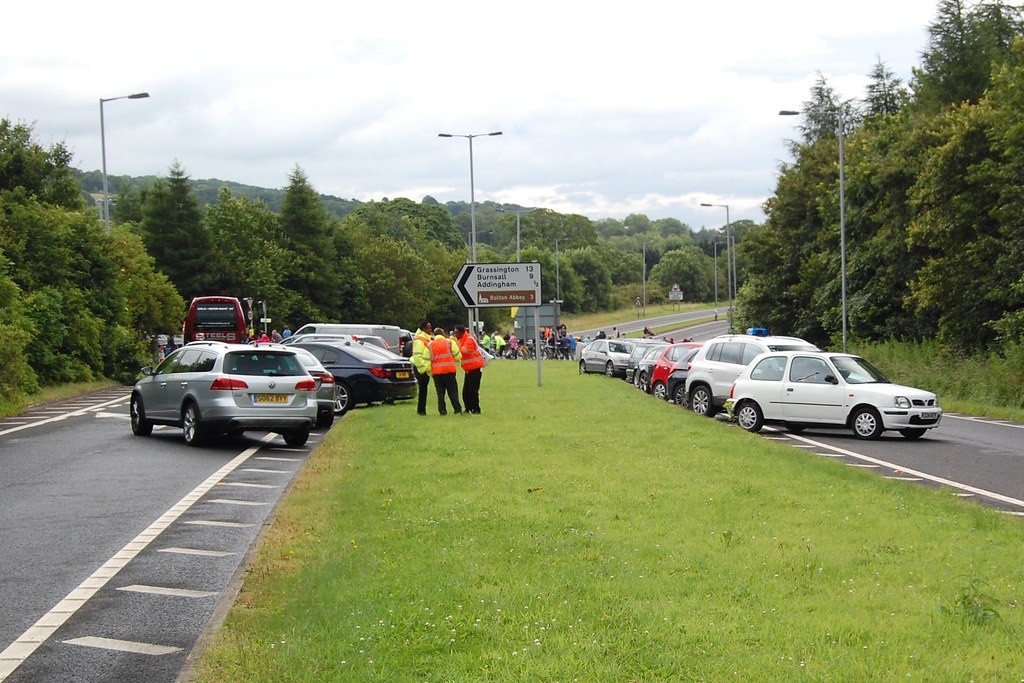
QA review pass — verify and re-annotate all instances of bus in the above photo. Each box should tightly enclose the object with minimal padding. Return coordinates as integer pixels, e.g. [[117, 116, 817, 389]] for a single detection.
[[183, 296, 247, 347]]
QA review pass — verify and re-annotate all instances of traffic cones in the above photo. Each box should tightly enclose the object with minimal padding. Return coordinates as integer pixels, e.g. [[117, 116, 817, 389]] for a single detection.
[[160, 345, 164, 362]]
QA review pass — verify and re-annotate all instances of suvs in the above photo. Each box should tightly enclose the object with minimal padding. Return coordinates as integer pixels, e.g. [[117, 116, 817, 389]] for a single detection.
[[685, 335, 824, 418], [129, 340, 319, 448]]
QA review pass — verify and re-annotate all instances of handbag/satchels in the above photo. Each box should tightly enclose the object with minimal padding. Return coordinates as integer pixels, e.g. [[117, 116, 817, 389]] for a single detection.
[[477, 346, 494, 364]]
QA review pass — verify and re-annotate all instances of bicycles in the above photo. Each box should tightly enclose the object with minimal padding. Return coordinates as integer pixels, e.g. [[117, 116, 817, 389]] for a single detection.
[[480, 339, 575, 361]]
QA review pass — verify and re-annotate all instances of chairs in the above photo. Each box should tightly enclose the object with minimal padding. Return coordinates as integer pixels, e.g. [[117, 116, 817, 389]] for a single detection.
[[237, 356, 278, 375]]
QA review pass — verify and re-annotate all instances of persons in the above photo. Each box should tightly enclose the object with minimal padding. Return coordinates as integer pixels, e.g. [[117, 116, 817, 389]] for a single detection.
[[409, 321, 486, 416], [577, 326, 620, 342], [449, 331, 576, 360], [245, 325, 291, 344]]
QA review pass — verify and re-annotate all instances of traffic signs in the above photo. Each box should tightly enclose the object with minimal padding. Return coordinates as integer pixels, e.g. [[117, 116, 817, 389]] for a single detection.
[[453, 263, 543, 309]]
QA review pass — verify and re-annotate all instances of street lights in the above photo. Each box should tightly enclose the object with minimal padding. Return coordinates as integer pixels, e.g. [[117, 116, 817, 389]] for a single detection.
[[554, 238, 568, 299], [631, 240, 654, 318], [701, 203, 733, 332], [503, 208, 536, 263], [721, 236, 736, 301], [778, 110, 848, 355], [438, 131, 503, 347], [714, 241, 727, 306], [100, 93, 151, 234]]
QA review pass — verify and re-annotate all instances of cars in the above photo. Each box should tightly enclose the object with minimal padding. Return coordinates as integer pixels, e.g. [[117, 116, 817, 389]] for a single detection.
[[577, 334, 702, 410], [728, 351, 945, 440], [145, 334, 183, 348], [232, 346, 335, 428], [279, 324, 417, 417]]
